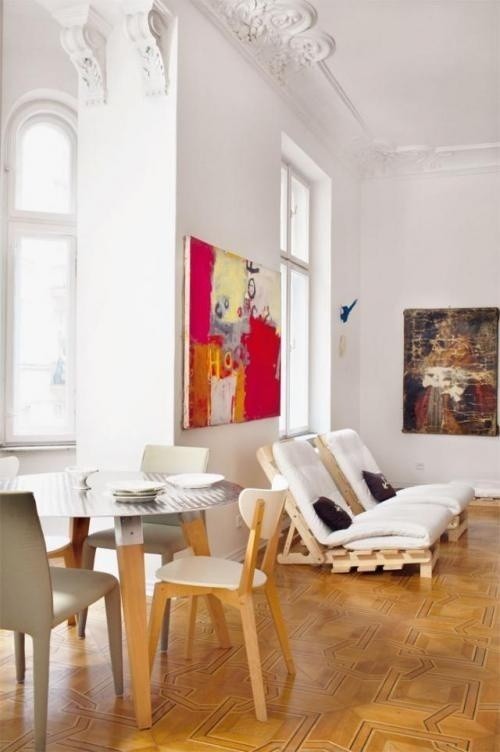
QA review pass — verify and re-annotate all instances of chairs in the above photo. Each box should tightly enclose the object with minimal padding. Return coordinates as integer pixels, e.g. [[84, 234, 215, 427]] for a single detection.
[[255, 427, 476, 578]]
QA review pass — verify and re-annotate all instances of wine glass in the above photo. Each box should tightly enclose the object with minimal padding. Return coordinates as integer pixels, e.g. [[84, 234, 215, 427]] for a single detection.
[[64, 465, 101, 490]]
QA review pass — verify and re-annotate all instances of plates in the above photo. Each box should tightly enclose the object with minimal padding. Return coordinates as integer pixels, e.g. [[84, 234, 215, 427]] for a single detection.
[[166, 472, 225, 489], [109, 479, 166, 504]]
